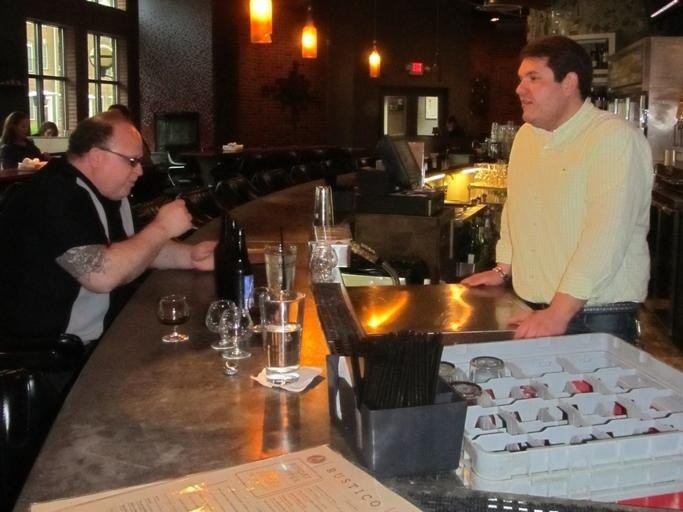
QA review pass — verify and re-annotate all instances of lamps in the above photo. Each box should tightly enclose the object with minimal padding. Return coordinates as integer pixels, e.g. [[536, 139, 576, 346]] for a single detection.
[[369, 40, 380, 78], [650, 0, 677, 17], [249, 0, 274, 45], [301, 1, 319, 60]]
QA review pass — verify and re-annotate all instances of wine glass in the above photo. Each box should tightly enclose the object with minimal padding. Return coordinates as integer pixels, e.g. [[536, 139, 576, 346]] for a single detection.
[[473, 162, 508, 189], [156, 294, 254, 361]]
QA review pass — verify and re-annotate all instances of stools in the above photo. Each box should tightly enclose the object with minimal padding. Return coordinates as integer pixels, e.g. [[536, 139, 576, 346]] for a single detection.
[[0, 148, 369, 512]]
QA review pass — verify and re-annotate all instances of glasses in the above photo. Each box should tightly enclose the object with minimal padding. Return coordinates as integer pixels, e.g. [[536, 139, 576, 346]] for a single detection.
[[92, 144, 142, 167]]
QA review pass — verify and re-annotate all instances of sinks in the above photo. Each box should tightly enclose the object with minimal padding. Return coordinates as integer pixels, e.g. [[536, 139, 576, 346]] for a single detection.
[[344, 272, 430, 286]]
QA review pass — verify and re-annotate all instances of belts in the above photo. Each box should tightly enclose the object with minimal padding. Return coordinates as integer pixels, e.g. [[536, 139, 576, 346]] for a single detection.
[[583, 301, 643, 313]]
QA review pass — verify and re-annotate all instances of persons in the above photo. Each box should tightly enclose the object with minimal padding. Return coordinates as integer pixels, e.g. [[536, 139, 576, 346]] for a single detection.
[[108, 104, 158, 201], [0, 111, 49, 170], [461, 36, 654, 339], [446, 116, 464, 150], [33, 120, 58, 137], [0, 109, 219, 397]]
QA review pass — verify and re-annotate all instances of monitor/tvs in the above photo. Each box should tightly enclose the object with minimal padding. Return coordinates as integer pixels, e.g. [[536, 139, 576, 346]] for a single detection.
[[382, 134, 421, 187]]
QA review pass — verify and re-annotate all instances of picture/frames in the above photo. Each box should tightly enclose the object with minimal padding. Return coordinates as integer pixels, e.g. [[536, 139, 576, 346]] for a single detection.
[[568, 33, 616, 75]]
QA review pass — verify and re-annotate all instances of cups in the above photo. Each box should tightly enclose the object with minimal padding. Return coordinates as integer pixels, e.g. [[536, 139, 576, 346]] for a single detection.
[[665, 149, 676, 166], [438, 356, 504, 405], [313, 184, 334, 229], [262, 391, 302, 464], [309, 243, 338, 284], [264, 243, 297, 299], [260, 290, 306, 383]]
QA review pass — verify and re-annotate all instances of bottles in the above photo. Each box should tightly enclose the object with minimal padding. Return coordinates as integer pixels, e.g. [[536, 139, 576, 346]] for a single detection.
[[590, 42, 608, 70], [213, 212, 255, 329]]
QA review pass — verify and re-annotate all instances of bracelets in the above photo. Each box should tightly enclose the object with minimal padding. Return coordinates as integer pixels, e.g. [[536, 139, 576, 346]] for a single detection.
[[492, 265, 509, 281]]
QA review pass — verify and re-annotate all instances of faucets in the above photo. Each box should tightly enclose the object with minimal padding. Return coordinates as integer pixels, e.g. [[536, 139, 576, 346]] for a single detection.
[[352, 244, 399, 286]]
